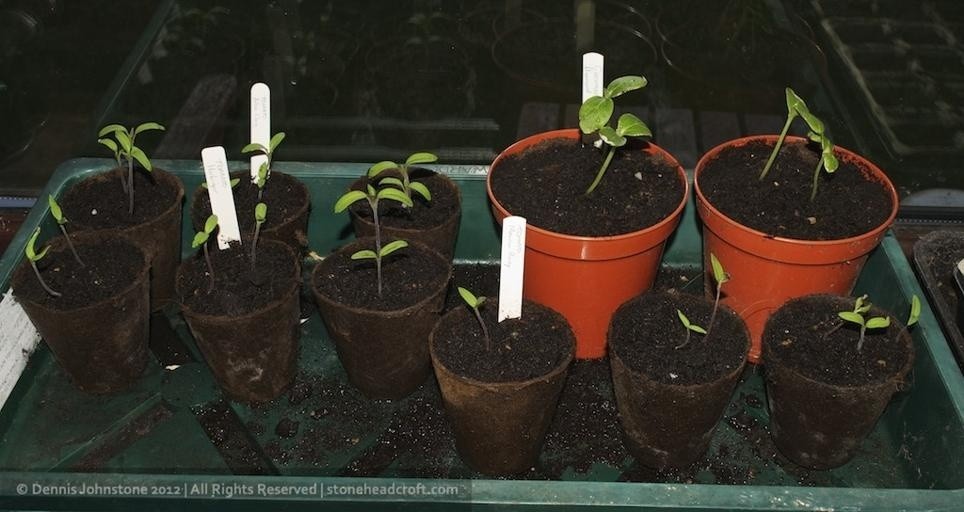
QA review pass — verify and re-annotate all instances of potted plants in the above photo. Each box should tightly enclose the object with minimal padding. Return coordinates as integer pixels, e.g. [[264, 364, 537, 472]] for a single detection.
[[190, 131, 312, 267], [693, 87, 899, 366], [59, 122, 186, 317], [10, 194, 154, 394], [309, 183, 453, 399], [608, 252, 754, 473], [345, 152, 461, 262], [172, 203, 302, 406], [426, 285, 578, 476], [763, 291, 927, 471], [485, 75, 689, 364]]
